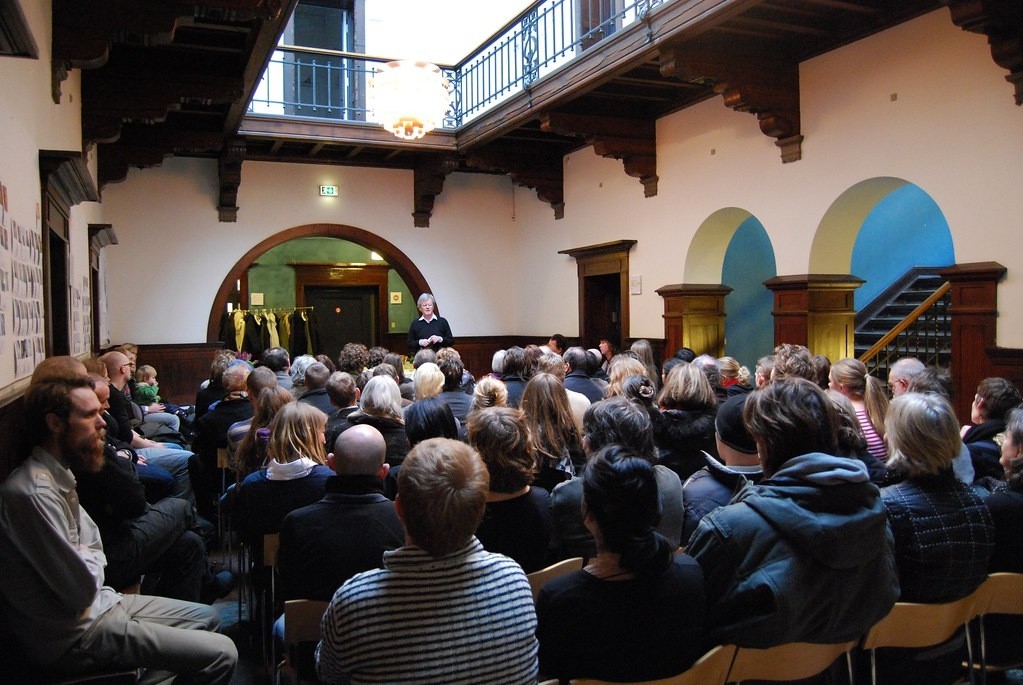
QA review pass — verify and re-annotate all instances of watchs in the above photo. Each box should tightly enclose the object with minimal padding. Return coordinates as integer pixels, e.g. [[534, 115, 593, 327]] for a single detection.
[[121, 449, 131, 459]]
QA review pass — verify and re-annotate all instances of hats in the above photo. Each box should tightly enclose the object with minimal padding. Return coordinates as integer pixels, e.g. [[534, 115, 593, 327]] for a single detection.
[[714, 393, 758, 455]]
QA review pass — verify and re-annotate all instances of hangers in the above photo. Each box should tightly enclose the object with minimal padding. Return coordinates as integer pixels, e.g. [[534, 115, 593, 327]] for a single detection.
[[244, 306, 296, 315]]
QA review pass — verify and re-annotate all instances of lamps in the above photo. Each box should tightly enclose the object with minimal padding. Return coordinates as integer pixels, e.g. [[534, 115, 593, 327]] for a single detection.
[[368, 58, 455, 140]]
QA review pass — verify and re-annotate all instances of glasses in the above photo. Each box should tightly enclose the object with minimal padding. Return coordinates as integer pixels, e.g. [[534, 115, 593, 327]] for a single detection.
[[887, 380, 900, 391], [420, 303, 433, 307], [123, 362, 132, 367]]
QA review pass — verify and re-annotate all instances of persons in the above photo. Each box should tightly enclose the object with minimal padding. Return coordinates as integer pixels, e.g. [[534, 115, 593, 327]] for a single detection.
[[406, 293, 455, 355], [313, 435, 540, 685], [0, 366, 239, 685], [0, 334, 1023, 685], [535, 444, 709, 685]]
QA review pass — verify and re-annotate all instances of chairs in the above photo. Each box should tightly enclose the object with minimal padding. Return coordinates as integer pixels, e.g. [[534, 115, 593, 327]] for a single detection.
[[527, 557, 583, 599], [725, 640, 857, 685], [212, 447, 230, 565], [570, 645, 726, 685], [259, 533, 281, 673], [962, 572, 1023, 685], [862, 579, 992, 685], [273, 598, 329, 685]]
